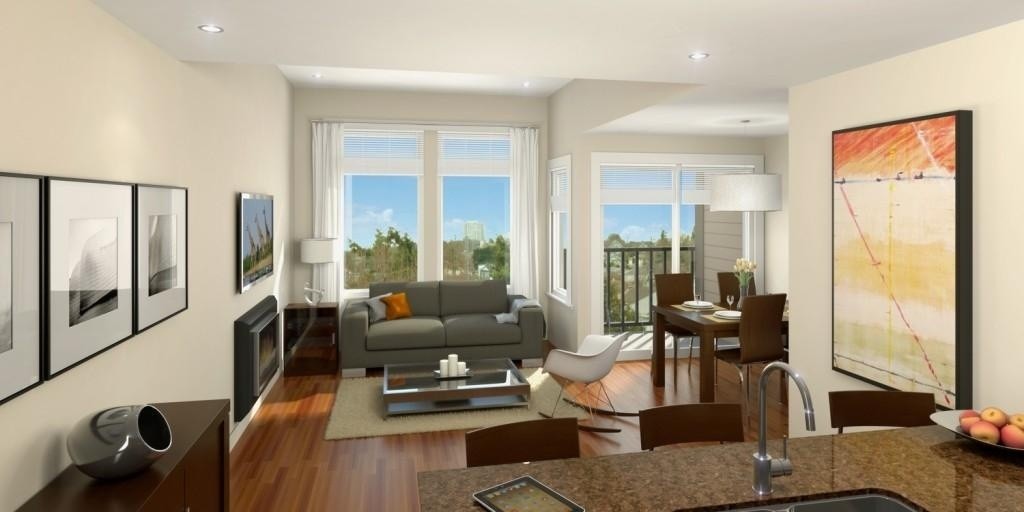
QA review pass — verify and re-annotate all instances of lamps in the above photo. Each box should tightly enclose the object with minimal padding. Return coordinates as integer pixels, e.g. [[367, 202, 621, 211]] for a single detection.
[[301, 239, 334, 307]]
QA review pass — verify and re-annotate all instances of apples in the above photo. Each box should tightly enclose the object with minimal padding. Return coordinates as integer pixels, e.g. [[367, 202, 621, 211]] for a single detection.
[[959, 407, 1024, 449]]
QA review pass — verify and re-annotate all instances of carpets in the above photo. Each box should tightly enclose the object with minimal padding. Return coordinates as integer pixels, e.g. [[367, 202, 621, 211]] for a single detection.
[[324, 367, 590, 440]]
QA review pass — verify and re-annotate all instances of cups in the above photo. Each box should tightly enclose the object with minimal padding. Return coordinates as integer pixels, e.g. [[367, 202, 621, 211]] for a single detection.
[[695, 295, 701, 305], [440, 354, 466, 377]]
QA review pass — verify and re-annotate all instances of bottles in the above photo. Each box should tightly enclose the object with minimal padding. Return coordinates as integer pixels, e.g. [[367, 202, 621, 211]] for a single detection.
[[64, 404, 173, 479]]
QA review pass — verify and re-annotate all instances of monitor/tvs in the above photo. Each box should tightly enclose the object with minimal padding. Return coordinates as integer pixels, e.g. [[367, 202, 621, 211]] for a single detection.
[[239, 191, 274, 297]]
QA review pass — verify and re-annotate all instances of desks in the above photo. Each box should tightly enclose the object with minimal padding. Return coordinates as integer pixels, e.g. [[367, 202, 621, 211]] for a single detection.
[[651, 301, 788, 402]]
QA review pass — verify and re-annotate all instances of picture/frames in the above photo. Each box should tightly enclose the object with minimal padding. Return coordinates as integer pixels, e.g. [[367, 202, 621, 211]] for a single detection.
[[252, 312, 282, 397], [832, 110, 973, 410]]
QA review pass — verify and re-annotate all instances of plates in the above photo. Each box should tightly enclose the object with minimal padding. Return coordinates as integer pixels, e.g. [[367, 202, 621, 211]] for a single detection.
[[434, 371, 473, 379], [929, 410, 1024, 451], [682, 301, 713, 309], [713, 311, 741, 320]]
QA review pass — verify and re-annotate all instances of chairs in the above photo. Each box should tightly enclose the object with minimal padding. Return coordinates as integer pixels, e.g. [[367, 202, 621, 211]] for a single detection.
[[828, 391, 936, 433], [718, 272, 756, 301], [538, 332, 640, 433], [714, 294, 789, 417], [466, 418, 580, 466], [651, 273, 718, 386], [639, 404, 744, 449]]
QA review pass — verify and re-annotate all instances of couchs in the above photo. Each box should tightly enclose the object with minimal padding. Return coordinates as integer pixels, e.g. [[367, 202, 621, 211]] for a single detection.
[[341, 280, 543, 378]]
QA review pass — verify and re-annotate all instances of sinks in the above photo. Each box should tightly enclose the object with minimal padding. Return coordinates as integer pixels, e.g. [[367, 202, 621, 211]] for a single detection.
[[652, 484, 934, 512]]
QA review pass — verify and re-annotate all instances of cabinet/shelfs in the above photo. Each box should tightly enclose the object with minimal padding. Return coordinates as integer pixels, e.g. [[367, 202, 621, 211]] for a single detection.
[[15, 400, 229, 512], [284, 302, 339, 378]]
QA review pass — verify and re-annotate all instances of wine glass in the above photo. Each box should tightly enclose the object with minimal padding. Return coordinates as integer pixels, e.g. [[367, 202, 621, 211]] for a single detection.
[[727, 295, 734, 311]]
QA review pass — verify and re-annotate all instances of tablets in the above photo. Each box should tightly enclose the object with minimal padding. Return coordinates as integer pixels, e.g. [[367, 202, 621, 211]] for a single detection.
[[472, 475, 586, 512]]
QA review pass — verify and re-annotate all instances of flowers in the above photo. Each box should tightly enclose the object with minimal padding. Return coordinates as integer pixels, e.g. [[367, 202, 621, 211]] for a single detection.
[[733, 258, 759, 286]]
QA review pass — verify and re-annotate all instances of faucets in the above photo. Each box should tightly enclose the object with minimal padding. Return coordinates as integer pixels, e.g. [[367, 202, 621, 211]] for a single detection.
[[744, 357, 819, 495]]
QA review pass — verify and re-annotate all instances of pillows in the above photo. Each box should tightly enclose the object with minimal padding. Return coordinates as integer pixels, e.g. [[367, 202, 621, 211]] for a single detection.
[[364, 280, 508, 323]]
